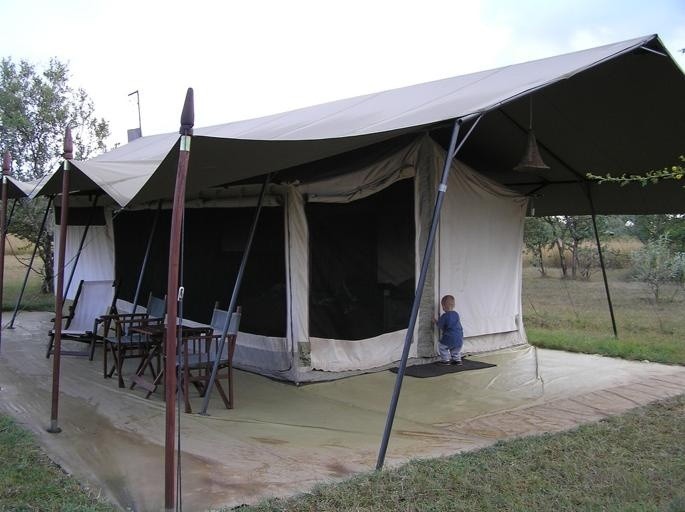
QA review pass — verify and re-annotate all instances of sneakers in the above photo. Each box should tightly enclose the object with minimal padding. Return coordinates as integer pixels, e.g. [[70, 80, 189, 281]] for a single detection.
[[437, 360, 463, 366]]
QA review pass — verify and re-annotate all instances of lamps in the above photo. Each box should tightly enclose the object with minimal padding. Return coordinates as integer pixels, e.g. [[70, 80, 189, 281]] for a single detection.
[[513, 95, 551, 173]]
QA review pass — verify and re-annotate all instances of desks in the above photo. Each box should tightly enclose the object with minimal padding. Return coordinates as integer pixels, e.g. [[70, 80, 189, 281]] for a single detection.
[[128, 325, 203, 399]]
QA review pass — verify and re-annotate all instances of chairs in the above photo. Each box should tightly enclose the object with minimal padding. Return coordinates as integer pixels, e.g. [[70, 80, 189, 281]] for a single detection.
[[100, 291, 167, 388], [164, 301, 241, 413], [46, 280, 120, 360]]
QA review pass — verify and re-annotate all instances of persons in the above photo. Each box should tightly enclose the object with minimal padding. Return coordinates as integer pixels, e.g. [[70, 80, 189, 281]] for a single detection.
[[431, 295, 464, 366]]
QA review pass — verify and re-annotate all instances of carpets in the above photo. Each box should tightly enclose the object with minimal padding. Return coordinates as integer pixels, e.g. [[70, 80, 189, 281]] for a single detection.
[[388, 358, 496, 378]]
